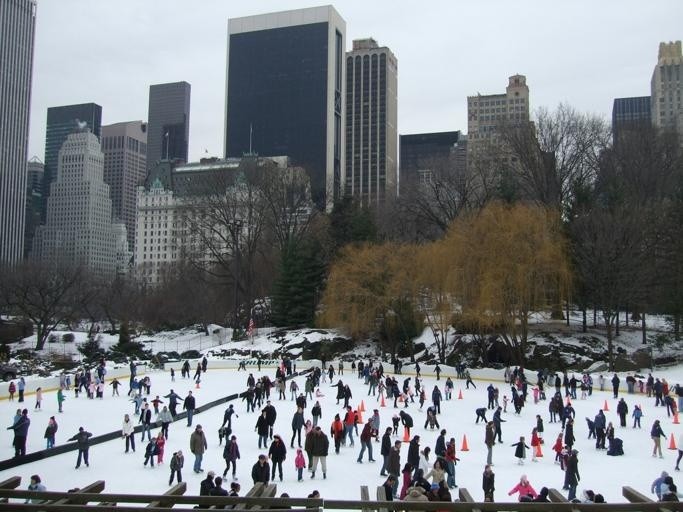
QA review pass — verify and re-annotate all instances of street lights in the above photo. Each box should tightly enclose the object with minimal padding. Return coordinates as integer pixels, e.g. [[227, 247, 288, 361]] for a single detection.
[[231, 218, 242, 341]]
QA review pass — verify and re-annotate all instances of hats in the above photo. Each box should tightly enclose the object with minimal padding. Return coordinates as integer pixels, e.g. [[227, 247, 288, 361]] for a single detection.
[[571, 449, 579, 456], [207, 471, 216, 476], [520, 474, 527, 483]]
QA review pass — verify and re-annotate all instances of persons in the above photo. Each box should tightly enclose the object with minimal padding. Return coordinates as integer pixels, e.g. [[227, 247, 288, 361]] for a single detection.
[[0, 352, 683, 512]]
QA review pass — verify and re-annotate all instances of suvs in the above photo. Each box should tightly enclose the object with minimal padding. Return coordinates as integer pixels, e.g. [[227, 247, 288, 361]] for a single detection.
[[0, 360, 17, 382]]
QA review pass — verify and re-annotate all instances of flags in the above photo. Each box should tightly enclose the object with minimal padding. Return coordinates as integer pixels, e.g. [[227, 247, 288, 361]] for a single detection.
[[245, 319, 254, 339]]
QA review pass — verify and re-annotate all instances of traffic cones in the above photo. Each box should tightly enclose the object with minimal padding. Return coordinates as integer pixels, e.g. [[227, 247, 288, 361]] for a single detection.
[[667, 432, 678, 450], [355, 391, 426, 443], [672, 412, 681, 424], [638, 404, 645, 417], [196, 382, 201, 388], [458, 388, 463, 399], [603, 399, 610, 411], [535, 441, 544, 457], [565, 394, 572, 405], [461, 433, 470, 451]]
